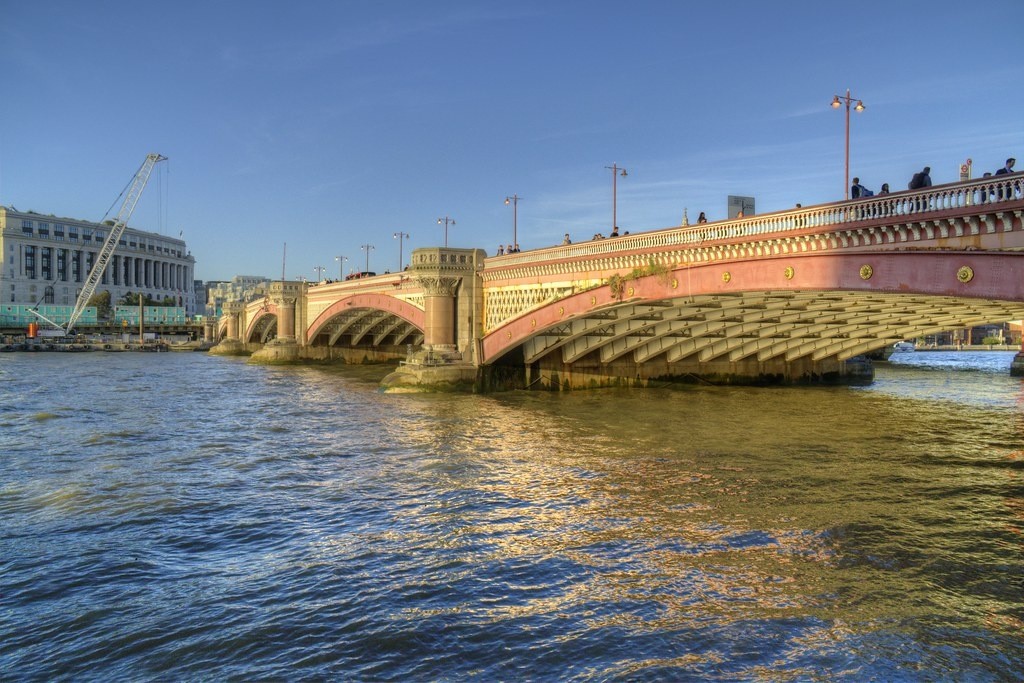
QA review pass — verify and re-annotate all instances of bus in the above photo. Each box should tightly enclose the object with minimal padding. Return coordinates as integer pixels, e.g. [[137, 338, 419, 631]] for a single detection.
[[988, 329, 1022, 342], [346, 272, 376, 281]]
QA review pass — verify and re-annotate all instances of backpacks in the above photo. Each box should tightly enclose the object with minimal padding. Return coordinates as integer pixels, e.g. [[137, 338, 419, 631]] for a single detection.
[[854, 185, 874, 198], [911, 172, 928, 189]]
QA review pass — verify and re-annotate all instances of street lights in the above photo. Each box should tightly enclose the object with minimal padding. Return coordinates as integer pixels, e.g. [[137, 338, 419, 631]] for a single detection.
[[831, 89, 869, 219], [335, 256, 348, 282], [361, 243, 375, 276], [438, 217, 455, 247], [394, 231, 409, 271], [296, 276, 305, 281], [604, 162, 628, 228], [314, 266, 326, 283], [505, 193, 522, 249]]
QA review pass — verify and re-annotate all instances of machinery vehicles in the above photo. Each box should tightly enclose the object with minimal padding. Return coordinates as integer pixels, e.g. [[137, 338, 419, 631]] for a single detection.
[[25, 153, 168, 338]]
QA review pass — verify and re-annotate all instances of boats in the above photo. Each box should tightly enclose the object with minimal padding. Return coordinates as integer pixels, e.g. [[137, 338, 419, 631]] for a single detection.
[[894, 343, 915, 353], [1010, 353, 1024, 377]]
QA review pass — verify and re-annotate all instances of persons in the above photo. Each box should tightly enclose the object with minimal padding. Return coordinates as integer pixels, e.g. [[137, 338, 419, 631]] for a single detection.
[[908, 167, 932, 211], [609, 227, 618, 237], [795, 204, 806, 226], [496, 245, 504, 256], [504, 245, 513, 255], [852, 177, 868, 217], [512, 244, 520, 253], [736, 211, 746, 235], [980, 172, 994, 203], [592, 233, 605, 240], [995, 158, 1021, 199], [878, 183, 894, 215], [326, 264, 409, 284], [564, 234, 571, 244], [697, 212, 708, 239]]
